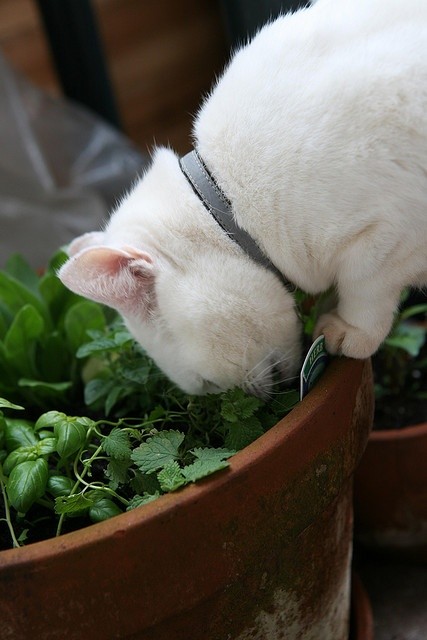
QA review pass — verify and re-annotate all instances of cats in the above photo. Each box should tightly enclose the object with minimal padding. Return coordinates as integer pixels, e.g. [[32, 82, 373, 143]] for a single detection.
[[54, 0, 427, 400]]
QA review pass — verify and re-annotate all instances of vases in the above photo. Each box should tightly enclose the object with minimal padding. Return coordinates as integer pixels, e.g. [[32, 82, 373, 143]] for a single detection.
[[291, 286, 427, 551]]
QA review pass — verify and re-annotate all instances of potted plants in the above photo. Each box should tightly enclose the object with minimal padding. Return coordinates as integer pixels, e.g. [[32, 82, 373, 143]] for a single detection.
[[0, 249, 427, 640]]
